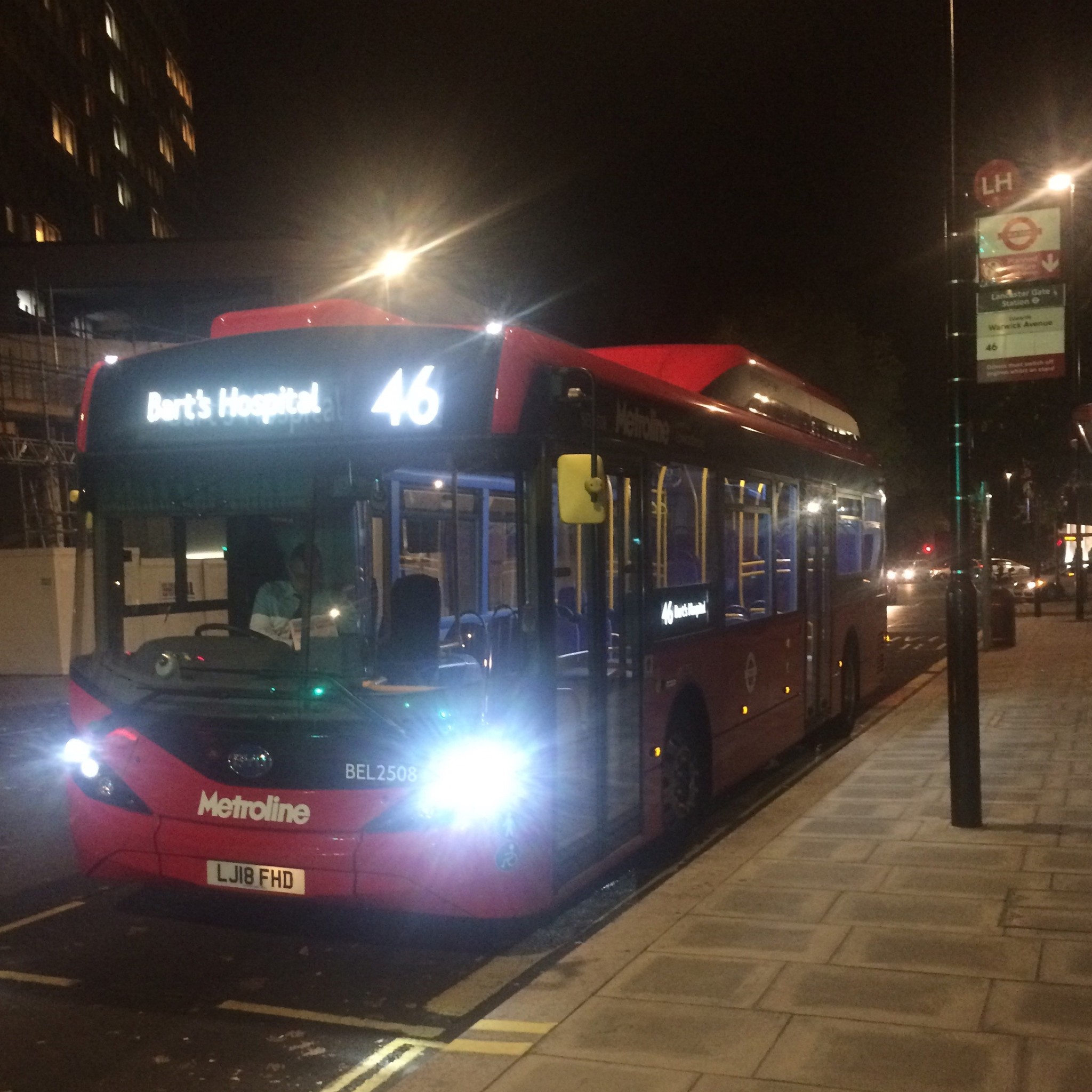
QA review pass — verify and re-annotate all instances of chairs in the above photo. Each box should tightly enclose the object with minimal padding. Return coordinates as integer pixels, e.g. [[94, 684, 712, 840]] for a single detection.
[[380, 526, 873, 690]]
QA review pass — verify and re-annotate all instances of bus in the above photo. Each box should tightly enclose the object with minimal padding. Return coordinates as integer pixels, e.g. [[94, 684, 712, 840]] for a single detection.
[[54, 297, 892, 937]]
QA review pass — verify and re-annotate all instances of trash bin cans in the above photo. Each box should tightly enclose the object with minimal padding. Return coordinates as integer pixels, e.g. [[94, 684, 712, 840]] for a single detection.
[[991, 586, 1017, 645]]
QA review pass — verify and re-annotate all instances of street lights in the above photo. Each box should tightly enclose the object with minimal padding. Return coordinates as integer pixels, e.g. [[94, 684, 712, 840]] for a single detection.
[[1046, 172, 1081, 408]]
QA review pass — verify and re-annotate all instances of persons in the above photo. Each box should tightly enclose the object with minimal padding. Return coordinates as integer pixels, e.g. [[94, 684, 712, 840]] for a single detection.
[[248, 541, 356, 653]]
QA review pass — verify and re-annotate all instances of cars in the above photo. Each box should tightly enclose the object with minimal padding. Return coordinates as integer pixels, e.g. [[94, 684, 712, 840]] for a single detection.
[[885, 557, 1065, 639]]
